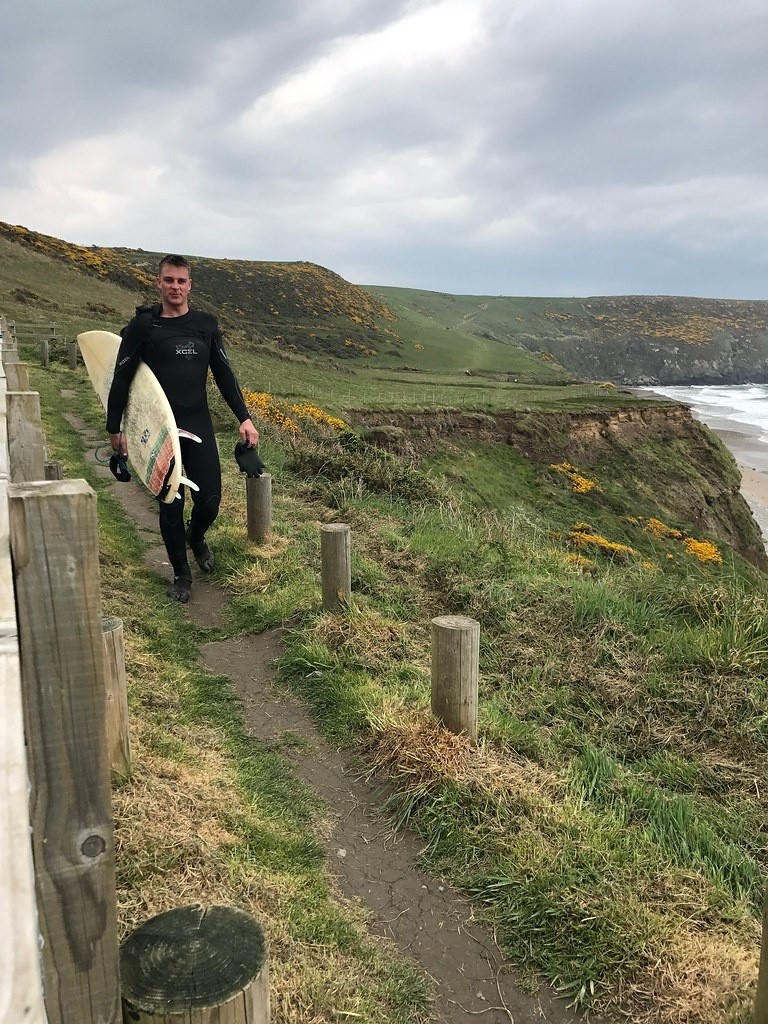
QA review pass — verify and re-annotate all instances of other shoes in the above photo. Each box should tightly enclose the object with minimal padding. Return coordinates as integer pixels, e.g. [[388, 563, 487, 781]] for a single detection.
[[169, 574, 193, 603], [185, 519, 215, 573]]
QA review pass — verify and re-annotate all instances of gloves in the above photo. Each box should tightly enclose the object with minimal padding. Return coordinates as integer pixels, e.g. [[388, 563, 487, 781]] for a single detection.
[[234, 439, 265, 479]]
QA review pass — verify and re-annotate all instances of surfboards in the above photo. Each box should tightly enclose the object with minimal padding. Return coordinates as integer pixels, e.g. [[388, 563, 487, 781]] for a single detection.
[[77, 329, 185, 504]]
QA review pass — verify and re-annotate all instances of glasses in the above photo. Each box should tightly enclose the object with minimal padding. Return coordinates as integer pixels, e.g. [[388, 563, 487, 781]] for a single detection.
[[110, 455, 131, 482]]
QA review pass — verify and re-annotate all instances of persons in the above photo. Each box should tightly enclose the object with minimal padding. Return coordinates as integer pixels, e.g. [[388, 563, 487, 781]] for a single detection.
[[105, 256, 260, 600]]
